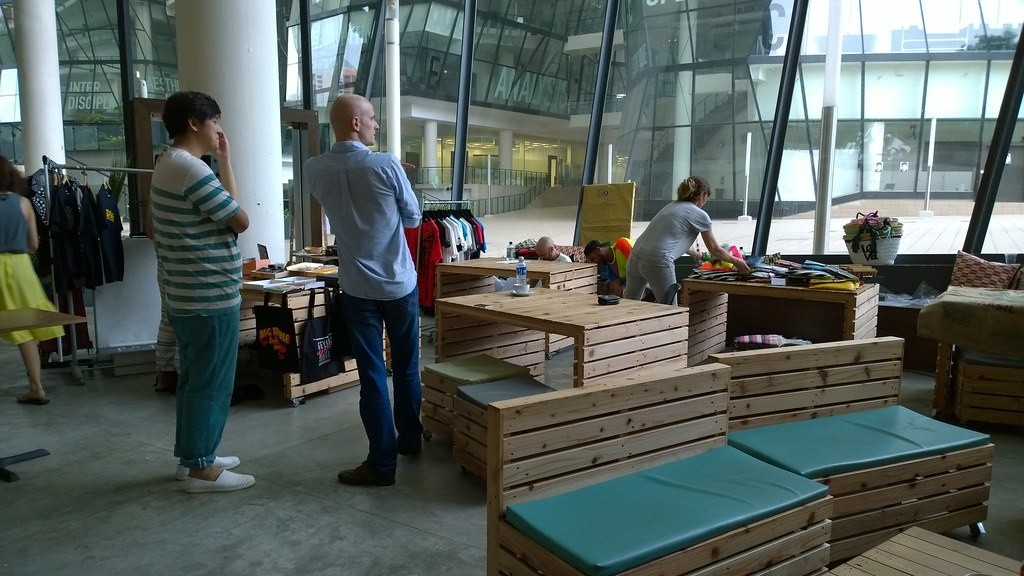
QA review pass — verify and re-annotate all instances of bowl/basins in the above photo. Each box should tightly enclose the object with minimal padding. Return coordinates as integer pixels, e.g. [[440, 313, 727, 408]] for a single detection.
[[514, 284, 531, 292]]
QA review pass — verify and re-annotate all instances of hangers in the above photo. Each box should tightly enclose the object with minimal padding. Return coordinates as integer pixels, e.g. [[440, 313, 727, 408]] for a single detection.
[[422, 200, 475, 220], [49, 159, 111, 189]]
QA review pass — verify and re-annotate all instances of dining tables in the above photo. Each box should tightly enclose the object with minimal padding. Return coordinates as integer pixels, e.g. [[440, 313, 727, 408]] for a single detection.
[[239, 289, 422, 407]]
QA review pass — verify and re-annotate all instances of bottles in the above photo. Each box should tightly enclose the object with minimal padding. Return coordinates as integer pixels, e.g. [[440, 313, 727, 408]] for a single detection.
[[516, 256, 527, 284], [506, 242, 516, 261], [739, 246, 746, 261]]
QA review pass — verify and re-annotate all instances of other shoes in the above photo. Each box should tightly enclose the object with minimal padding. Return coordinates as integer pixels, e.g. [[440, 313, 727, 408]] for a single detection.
[[177, 456, 241, 480], [185, 468, 255, 493]]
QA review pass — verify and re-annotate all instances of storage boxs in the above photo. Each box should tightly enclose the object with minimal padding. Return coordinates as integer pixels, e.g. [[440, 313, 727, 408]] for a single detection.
[[243, 257, 339, 290]]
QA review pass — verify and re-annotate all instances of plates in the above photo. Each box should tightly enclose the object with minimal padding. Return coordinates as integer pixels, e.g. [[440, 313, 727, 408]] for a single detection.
[[510, 290, 535, 296]]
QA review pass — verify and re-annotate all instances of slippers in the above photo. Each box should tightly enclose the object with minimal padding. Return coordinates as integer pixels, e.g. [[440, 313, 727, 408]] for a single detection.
[[16, 392, 50, 405]]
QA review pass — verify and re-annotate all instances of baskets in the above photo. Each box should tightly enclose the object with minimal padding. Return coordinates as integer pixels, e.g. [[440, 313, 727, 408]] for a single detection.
[[842, 209, 904, 265]]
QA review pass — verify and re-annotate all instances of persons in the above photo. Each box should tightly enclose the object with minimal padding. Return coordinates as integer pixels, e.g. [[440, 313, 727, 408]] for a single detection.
[[535, 236, 572, 288], [0, 155, 66, 405], [584, 237, 657, 303], [150, 91, 256, 493], [626, 176, 750, 307], [303, 94, 422, 487]]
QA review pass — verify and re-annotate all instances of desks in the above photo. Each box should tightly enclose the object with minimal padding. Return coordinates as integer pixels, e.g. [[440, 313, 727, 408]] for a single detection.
[[435, 288, 689, 388], [682, 278, 880, 367], [0, 307, 89, 483], [821, 525, 1023, 575], [435, 257, 598, 360], [917, 286, 1024, 427]]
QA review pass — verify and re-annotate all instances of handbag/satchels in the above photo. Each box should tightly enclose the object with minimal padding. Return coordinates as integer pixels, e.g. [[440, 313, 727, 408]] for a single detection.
[[332, 291, 357, 362], [300, 288, 345, 385], [254, 289, 300, 375]]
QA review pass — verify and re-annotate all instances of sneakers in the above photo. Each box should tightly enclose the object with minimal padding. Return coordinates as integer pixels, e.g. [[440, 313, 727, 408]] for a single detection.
[[338, 464, 396, 486], [397, 434, 422, 455]]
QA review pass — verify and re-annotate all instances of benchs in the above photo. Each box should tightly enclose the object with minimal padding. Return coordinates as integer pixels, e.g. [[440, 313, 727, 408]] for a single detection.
[[708, 337, 995, 561], [487, 363, 833, 576]]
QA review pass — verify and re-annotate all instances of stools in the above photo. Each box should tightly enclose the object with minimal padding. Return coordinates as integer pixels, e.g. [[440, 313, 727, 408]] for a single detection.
[[453, 376, 558, 492], [421, 353, 530, 441]]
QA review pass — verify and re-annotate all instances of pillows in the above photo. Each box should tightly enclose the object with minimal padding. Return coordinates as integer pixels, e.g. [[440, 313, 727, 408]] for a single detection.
[[950, 249, 1020, 288]]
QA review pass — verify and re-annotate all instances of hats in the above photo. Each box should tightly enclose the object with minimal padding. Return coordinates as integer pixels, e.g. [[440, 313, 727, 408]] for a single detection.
[[584, 239, 611, 257]]
